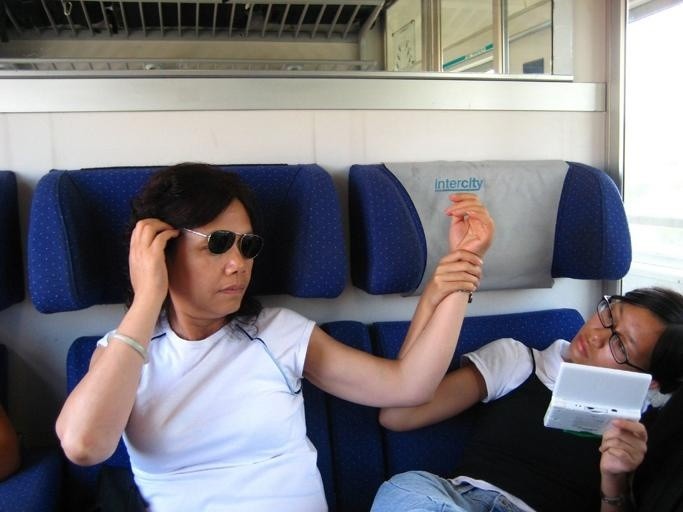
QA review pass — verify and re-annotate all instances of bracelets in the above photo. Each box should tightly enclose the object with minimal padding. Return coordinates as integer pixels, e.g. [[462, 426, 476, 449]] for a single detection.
[[457, 289, 473, 303], [599, 488, 633, 506], [112, 334, 148, 362]]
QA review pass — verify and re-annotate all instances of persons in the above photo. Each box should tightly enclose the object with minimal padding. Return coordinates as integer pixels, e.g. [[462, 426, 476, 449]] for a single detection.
[[0, 405, 21, 481], [55, 163, 495, 512], [370, 250, 683, 512]]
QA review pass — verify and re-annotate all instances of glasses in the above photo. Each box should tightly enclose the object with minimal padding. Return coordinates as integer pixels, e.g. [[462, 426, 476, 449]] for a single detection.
[[597, 295, 651, 373], [180, 228, 265, 259]]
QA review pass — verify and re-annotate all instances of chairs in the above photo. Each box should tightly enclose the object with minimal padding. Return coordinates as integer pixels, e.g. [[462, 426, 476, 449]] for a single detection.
[[25, 162, 385, 512], [349, 162, 637, 512]]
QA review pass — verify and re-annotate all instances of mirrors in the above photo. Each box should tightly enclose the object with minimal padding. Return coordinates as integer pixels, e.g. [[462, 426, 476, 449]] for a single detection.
[[0, 2, 572, 79]]
